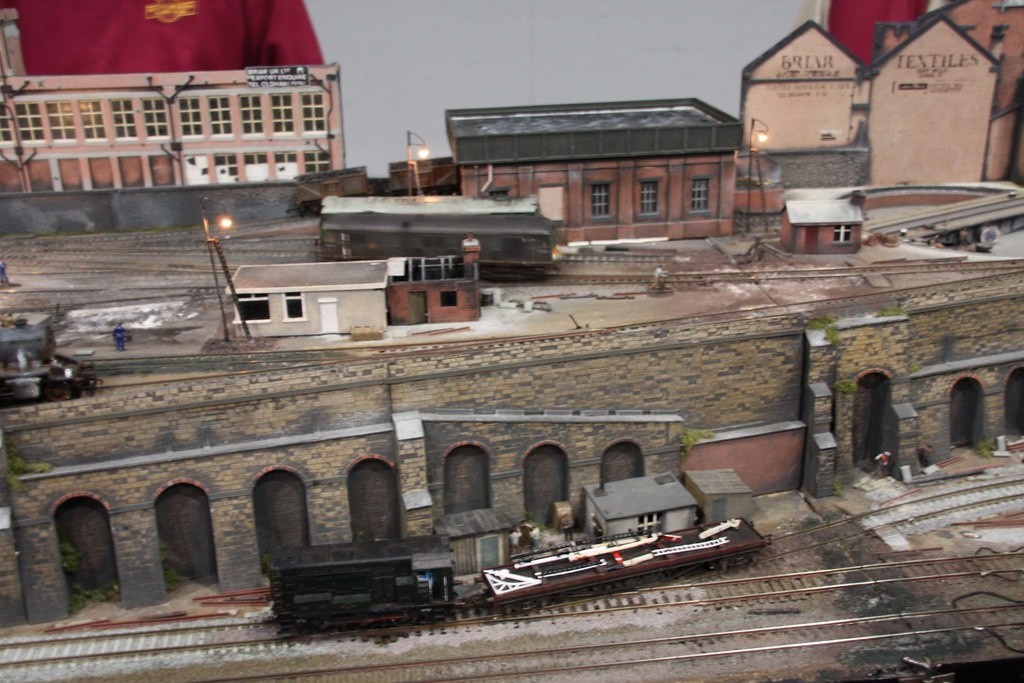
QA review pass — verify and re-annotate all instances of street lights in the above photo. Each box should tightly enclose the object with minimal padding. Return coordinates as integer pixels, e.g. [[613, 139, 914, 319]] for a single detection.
[[745, 116, 769, 232], [406, 128, 430, 196], [197, 196, 235, 344]]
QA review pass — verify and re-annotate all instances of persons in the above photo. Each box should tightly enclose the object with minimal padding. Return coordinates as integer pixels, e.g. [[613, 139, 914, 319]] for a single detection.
[[529, 524, 540, 549], [650, 265, 669, 290], [874, 451, 891, 480], [560, 513, 573, 540], [917, 444, 933, 466], [508, 529, 522, 554], [0, 258, 8, 283], [462, 232, 479, 246], [112, 323, 126, 351]]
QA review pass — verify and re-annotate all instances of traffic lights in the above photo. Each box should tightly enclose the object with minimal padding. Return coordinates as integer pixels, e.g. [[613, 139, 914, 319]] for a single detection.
[[314, 239, 323, 245]]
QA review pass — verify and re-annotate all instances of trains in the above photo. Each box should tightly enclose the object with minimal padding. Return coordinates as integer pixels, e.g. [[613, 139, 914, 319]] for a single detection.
[[308, 196, 556, 282], [0, 323, 104, 405], [268, 514, 772, 633]]
